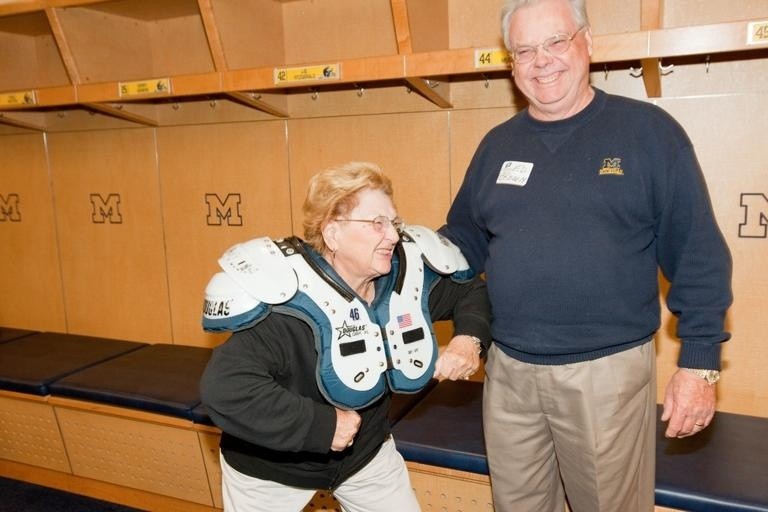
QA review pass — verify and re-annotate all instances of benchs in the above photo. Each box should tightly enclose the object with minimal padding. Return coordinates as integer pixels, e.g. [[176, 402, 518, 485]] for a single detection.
[[1, 326, 768, 512]]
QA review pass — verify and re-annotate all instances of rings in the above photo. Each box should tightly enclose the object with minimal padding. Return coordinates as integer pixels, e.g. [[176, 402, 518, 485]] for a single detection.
[[696, 423, 704, 427], [347, 440, 354, 447], [465, 377, 469, 380]]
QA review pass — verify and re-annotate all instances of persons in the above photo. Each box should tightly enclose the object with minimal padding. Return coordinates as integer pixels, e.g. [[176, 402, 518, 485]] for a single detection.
[[437, 0, 737, 512], [195, 160, 491, 512]]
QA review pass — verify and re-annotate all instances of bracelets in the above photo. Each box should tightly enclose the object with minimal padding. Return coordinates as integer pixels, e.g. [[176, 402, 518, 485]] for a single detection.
[[471, 335, 483, 354]]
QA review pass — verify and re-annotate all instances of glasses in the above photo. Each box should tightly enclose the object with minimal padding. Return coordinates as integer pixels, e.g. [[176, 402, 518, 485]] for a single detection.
[[339, 216, 405, 235], [508, 25, 586, 64]]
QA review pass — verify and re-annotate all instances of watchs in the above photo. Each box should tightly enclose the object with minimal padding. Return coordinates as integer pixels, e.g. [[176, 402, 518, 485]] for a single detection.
[[684, 368, 720, 385]]
